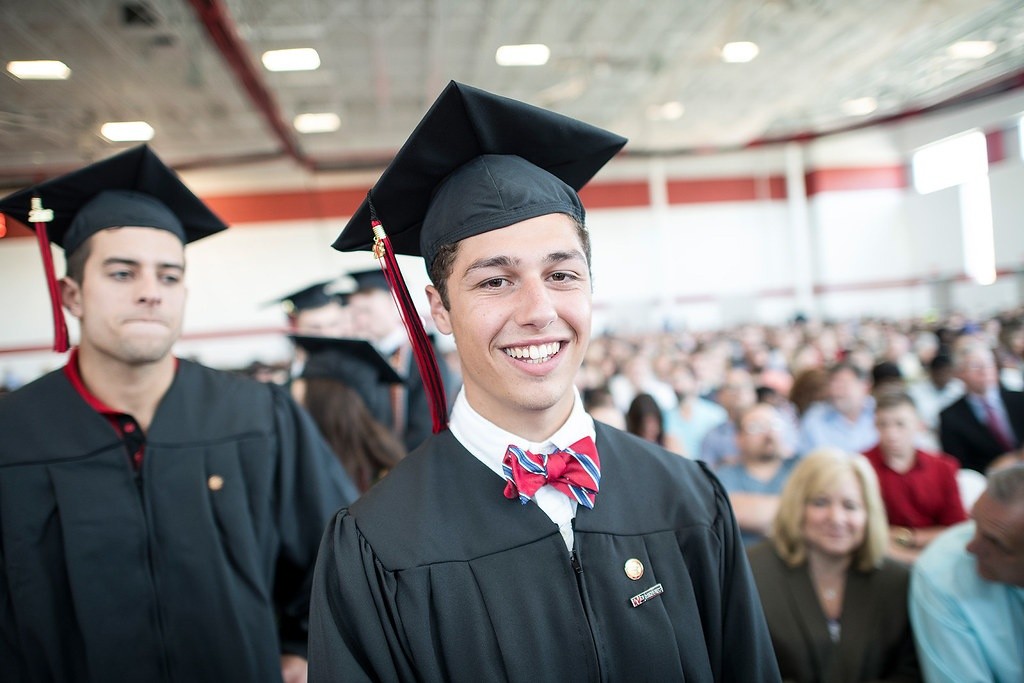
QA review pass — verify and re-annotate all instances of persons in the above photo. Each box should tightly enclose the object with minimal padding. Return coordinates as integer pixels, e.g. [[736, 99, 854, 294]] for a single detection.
[[908, 464, 1024, 683], [797, 362, 879, 454], [304, 80, 785, 683], [0, 140, 361, 683], [860, 393, 970, 549], [576, 313, 1024, 457], [659, 363, 728, 461], [746, 448, 925, 683], [244, 266, 462, 493], [937, 334, 1024, 475], [714, 402, 806, 546]]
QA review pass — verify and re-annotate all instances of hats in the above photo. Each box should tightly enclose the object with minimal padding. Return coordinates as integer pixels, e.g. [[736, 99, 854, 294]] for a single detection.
[[1, 140, 231, 354], [278, 260, 398, 314], [331, 78, 630, 435], [285, 333, 407, 409]]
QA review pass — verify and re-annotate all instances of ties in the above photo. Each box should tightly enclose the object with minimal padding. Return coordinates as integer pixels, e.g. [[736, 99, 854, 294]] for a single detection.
[[985, 400, 1011, 450]]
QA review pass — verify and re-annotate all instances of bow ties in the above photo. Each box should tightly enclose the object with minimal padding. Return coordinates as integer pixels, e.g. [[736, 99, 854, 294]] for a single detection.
[[501, 435, 603, 510]]
